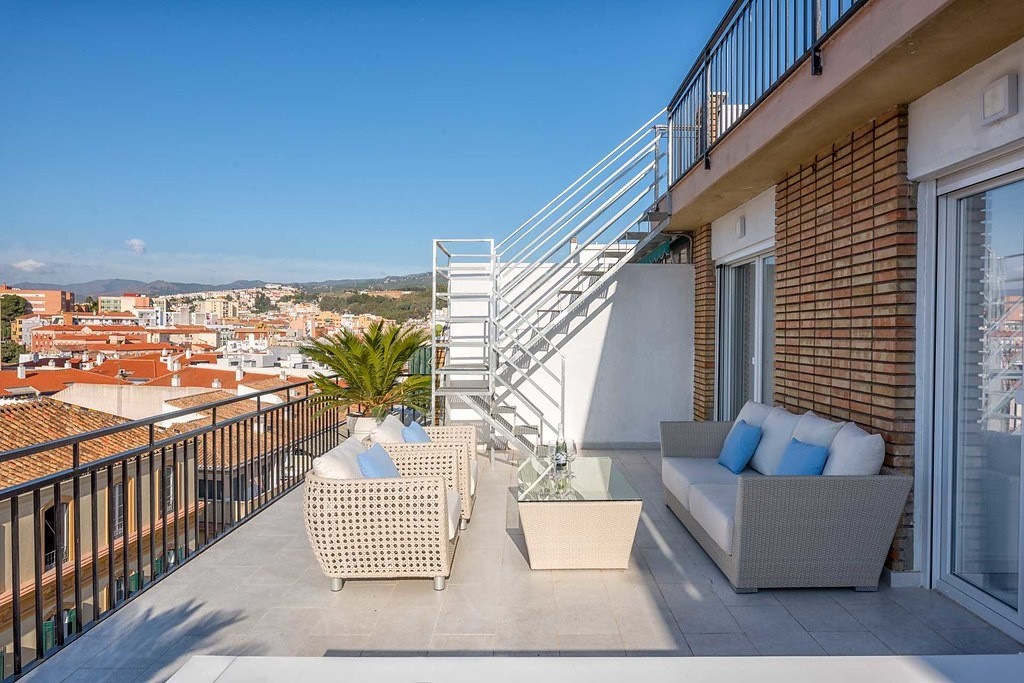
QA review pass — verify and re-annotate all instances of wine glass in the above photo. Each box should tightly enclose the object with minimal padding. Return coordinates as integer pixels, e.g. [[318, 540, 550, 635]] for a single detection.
[[566, 439, 577, 478], [548, 440, 556, 477]]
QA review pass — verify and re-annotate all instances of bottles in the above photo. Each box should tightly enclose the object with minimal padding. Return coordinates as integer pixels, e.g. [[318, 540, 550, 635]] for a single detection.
[[556, 424, 567, 470]]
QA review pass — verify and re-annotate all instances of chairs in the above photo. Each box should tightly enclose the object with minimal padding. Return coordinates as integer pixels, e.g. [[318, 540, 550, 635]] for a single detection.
[[362, 423, 478, 529], [304, 445, 458, 590]]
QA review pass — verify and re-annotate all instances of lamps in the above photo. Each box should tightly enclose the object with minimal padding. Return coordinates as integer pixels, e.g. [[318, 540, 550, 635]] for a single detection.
[[734, 217, 745, 238], [980, 72, 1018, 125]]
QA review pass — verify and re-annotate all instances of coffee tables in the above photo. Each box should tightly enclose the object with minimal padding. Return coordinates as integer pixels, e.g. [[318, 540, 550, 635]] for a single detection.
[[517, 455, 643, 570]]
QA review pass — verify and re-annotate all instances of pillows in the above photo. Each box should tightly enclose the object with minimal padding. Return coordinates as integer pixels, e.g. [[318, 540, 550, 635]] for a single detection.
[[792, 410, 847, 450], [357, 442, 401, 478], [402, 420, 430, 442], [822, 423, 886, 475], [729, 399, 774, 440], [749, 406, 803, 474], [370, 414, 407, 443], [312, 436, 362, 480], [719, 419, 762, 474], [776, 436, 829, 475]]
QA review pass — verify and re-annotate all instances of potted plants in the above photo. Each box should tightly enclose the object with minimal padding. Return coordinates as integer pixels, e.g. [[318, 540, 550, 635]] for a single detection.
[[295, 316, 450, 439]]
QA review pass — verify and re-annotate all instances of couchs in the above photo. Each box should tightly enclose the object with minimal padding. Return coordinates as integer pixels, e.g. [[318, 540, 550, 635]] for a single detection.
[[659, 420, 915, 594]]
